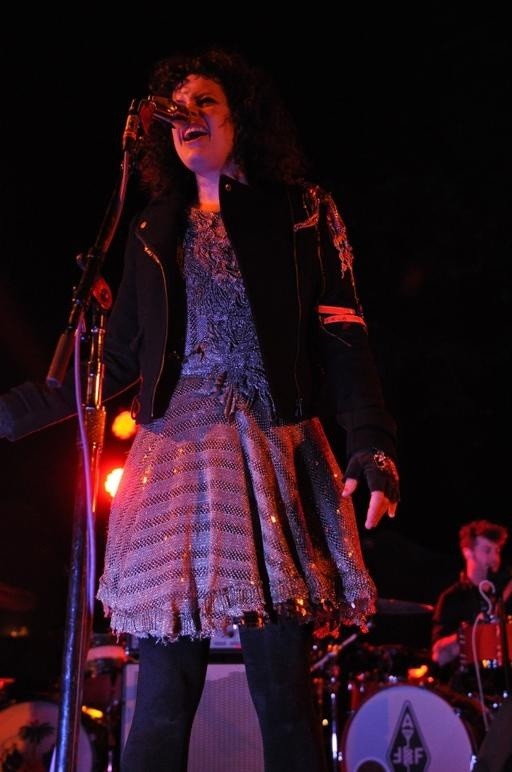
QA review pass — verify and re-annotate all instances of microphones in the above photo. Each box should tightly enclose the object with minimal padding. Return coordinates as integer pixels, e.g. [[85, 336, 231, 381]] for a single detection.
[[146, 94, 190, 129]]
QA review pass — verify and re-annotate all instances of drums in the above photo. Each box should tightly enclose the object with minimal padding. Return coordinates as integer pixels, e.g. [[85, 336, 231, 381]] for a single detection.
[[338, 676, 494, 772], [0, 700, 95, 772], [460, 614, 512, 675]]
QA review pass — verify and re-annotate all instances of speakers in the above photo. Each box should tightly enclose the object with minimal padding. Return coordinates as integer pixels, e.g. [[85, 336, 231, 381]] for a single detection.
[[119, 658, 265, 772]]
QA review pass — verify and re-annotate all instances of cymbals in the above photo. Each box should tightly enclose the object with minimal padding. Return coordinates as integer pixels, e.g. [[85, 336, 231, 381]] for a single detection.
[[376, 598, 435, 613]]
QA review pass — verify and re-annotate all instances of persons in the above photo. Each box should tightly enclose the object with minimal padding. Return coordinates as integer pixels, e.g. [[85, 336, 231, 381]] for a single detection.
[[0, 46, 402, 772], [33, 659, 65, 701], [432, 520, 509, 684]]
